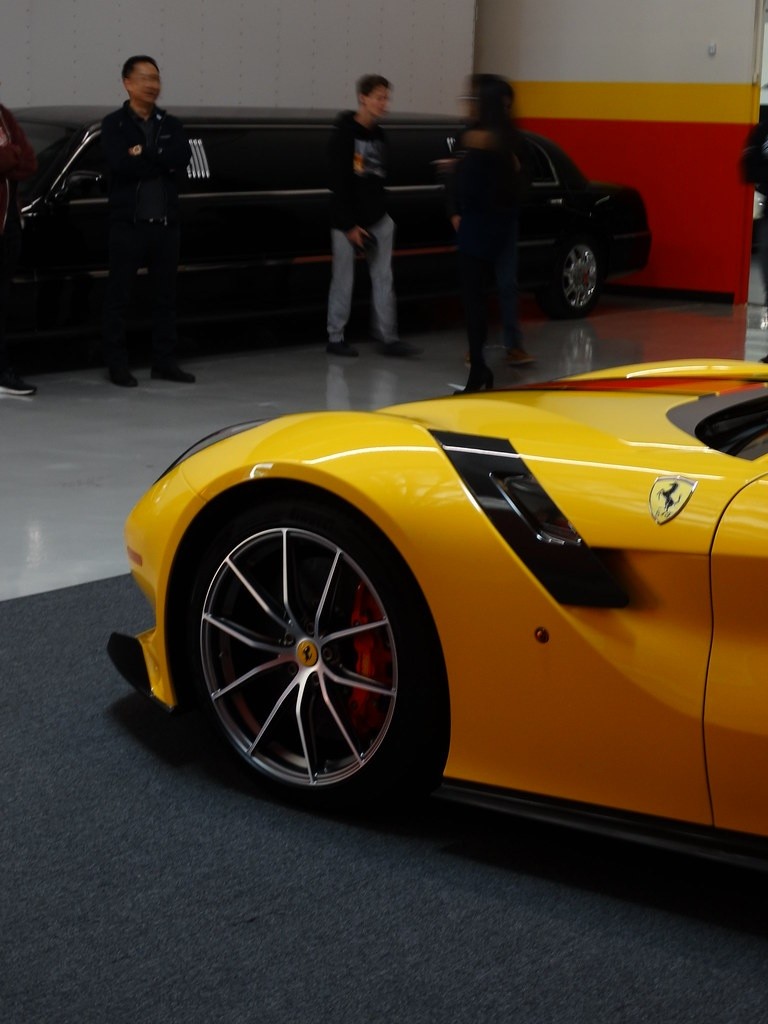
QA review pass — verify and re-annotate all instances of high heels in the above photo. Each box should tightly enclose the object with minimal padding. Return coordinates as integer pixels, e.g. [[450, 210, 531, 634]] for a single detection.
[[454, 369, 495, 394]]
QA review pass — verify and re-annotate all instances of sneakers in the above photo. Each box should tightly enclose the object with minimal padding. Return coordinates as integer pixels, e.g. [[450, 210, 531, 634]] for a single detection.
[[0, 371, 37, 395], [507, 348, 534, 365]]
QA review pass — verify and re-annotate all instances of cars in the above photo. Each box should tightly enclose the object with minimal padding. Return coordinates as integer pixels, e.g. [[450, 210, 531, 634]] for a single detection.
[[0, 101, 652, 319]]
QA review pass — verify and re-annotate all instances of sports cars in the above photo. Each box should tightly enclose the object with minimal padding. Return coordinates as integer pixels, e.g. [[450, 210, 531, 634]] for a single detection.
[[105, 353, 767, 872]]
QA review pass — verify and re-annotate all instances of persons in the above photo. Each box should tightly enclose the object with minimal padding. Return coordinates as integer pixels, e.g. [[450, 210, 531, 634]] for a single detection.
[[740, 118, 768, 365], [0, 103, 38, 394], [325, 73, 423, 357], [430, 74, 535, 395], [97, 56, 197, 386]]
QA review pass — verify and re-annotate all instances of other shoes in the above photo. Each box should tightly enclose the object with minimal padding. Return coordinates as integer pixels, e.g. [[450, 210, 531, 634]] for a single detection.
[[327, 340, 359, 356], [111, 370, 137, 387], [380, 340, 418, 355], [151, 365, 195, 382]]
